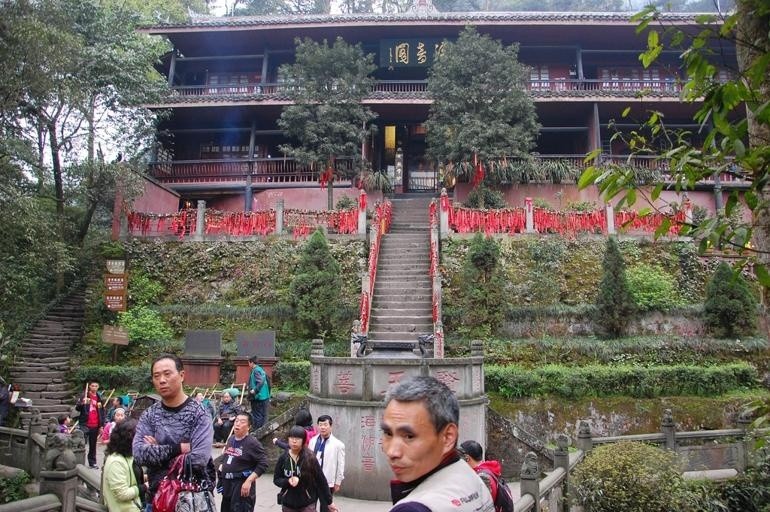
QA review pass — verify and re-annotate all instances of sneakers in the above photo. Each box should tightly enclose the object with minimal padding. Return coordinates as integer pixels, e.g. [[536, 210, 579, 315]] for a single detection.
[[89, 463, 99, 469]]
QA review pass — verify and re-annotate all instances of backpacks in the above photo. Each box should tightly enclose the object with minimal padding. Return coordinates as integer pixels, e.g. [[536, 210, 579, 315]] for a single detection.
[[477, 469, 514, 512]]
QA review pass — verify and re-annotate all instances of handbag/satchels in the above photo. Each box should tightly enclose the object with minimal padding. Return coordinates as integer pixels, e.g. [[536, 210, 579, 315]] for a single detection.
[[277, 489, 283, 504], [151, 453, 218, 512]]
